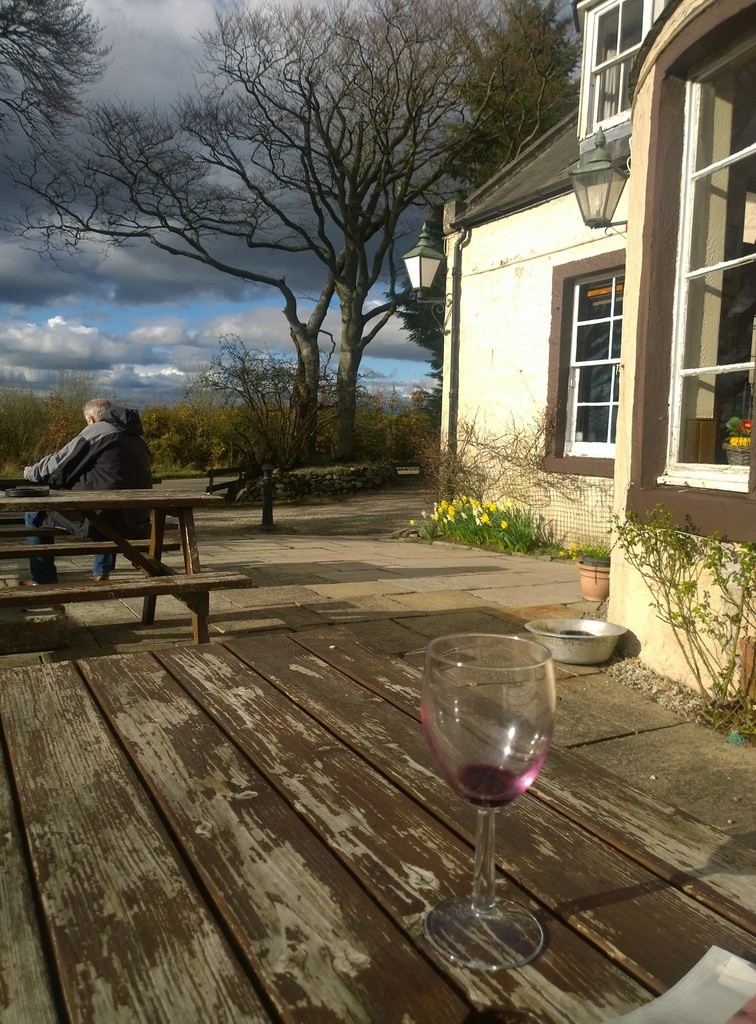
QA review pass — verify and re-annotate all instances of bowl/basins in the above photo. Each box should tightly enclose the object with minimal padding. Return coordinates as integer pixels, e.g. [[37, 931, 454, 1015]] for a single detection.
[[524, 619, 628, 664]]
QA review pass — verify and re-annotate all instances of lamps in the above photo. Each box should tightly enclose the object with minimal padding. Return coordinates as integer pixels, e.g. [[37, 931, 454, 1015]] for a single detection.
[[398, 221, 452, 336], [569, 121, 629, 235]]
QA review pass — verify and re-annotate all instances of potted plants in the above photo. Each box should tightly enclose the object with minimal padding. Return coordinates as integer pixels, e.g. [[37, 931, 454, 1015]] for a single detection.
[[579, 533, 614, 568]]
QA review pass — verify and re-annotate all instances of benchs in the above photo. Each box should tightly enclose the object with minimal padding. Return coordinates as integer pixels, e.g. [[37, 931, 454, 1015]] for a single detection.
[[0, 571, 252, 608], [0, 536, 181, 559], [0, 521, 179, 535]]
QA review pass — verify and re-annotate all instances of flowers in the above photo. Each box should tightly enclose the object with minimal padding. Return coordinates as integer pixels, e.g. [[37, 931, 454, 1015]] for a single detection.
[[724, 415, 752, 444]]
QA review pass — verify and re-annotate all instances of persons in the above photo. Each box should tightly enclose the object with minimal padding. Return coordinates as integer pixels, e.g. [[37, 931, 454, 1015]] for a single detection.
[[20, 398, 155, 586]]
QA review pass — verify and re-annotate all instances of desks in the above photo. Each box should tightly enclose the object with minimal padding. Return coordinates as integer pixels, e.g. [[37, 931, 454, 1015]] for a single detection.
[[0, 485, 223, 646], [0, 475, 163, 489], [0, 627, 756, 1024]]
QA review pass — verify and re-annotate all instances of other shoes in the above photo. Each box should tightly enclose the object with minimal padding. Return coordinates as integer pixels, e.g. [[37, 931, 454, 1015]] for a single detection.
[[19, 580, 40, 586], [90, 576, 109, 582]]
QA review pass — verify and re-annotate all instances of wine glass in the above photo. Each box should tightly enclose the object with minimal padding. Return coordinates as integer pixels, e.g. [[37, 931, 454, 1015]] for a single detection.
[[420, 633, 556, 970]]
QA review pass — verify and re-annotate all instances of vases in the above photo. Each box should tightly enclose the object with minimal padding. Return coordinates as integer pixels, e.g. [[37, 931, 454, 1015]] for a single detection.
[[576, 561, 610, 602], [722, 443, 751, 466]]
